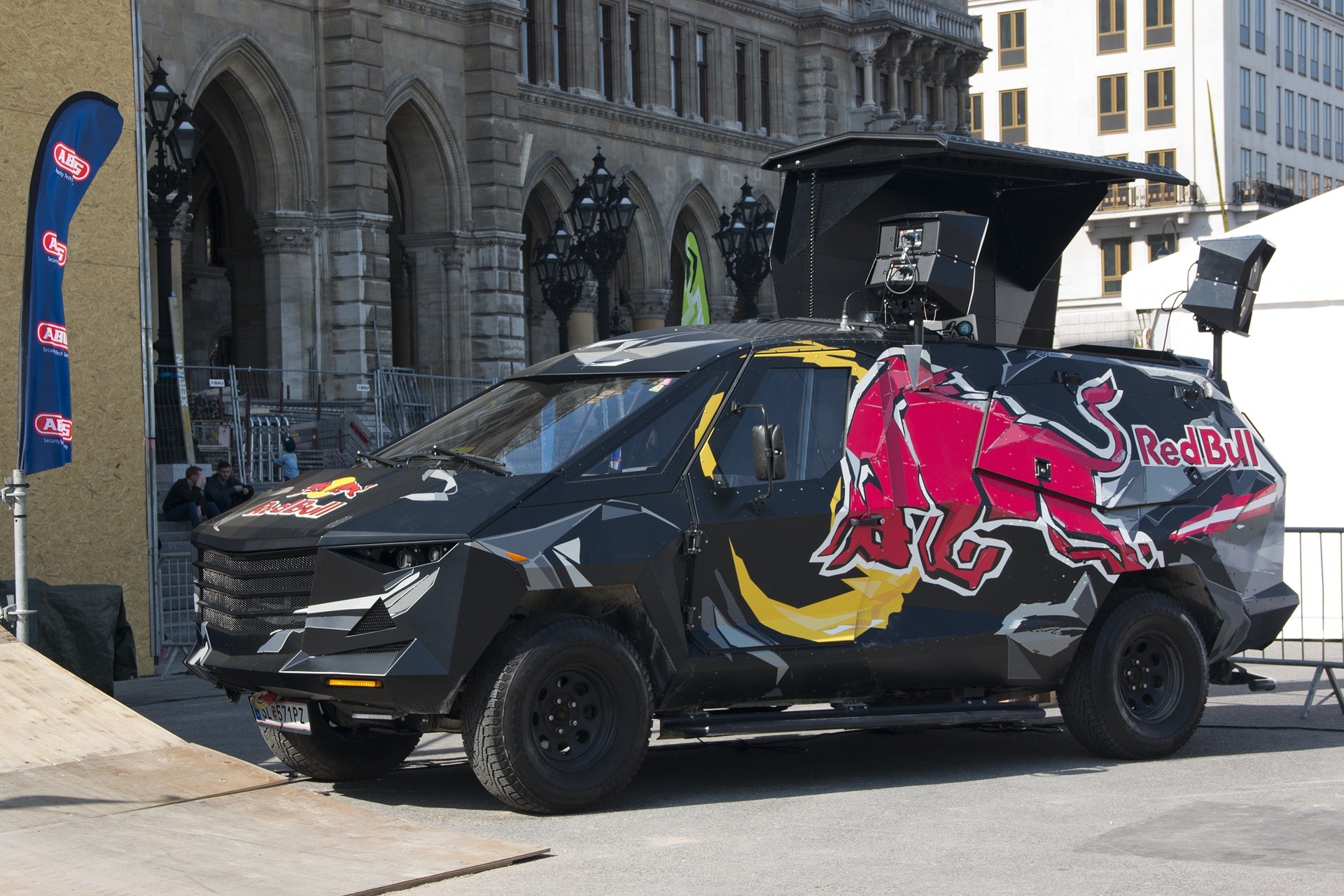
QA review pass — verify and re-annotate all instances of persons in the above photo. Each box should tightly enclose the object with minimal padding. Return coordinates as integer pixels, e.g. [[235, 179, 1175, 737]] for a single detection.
[[270, 437, 300, 482], [204, 460, 254, 513], [161, 466, 221, 529]]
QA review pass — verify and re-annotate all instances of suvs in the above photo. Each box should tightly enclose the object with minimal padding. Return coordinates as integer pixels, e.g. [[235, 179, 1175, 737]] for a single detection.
[[196, 132, 1303, 812]]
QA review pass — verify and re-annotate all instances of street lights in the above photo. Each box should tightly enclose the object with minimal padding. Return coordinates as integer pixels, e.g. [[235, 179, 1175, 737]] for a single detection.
[[530, 211, 588, 358], [713, 174, 778, 322], [564, 146, 638, 343]]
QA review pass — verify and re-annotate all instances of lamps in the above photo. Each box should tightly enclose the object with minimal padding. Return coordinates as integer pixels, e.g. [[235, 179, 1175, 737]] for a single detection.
[[864, 211, 989, 317], [1184, 235, 1275, 379]]
